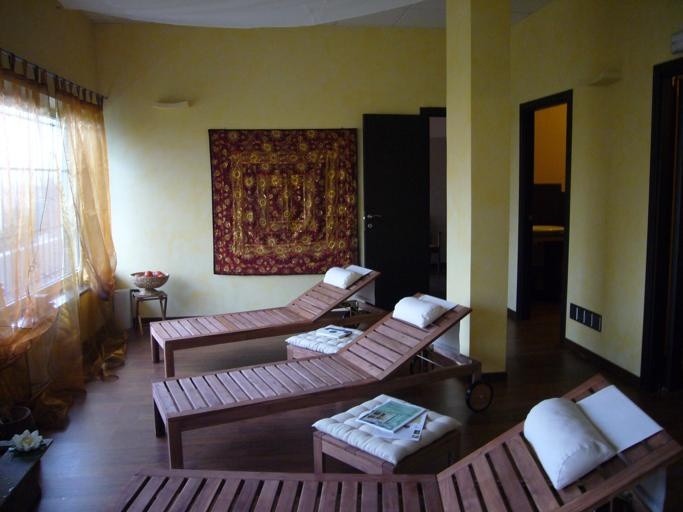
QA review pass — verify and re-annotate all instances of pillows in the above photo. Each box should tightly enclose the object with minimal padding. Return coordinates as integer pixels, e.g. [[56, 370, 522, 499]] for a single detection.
[[524, 398, 616, 490], [323, 266, 362, 289], [392, 297, 448, 328]]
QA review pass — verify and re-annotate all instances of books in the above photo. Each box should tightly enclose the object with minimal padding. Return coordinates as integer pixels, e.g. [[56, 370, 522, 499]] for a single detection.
[[316, 326, 351, 338], [358, 398, 426, 433]]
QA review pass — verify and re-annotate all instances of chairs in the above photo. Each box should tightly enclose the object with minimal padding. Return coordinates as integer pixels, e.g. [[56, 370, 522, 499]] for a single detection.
[[103, 376, 683, 511], [150, 265, 380, 377], [153, 293, 481, 469]]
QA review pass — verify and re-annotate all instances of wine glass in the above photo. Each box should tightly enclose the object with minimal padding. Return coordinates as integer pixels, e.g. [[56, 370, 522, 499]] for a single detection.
[[129, 272, 169, 297]]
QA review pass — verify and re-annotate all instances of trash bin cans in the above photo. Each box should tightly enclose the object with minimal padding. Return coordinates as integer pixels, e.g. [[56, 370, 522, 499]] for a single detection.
[[0, 406, 35, 441]]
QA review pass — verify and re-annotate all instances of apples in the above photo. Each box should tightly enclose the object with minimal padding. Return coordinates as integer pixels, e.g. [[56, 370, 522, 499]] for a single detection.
[[144, 270, 164, 277]]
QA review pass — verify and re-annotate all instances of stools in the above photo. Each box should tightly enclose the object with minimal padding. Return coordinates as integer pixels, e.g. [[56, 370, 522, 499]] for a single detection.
[[135, 292, 167, 335], [313, 395, 462, 475], [286, 325, 364, 360]]
